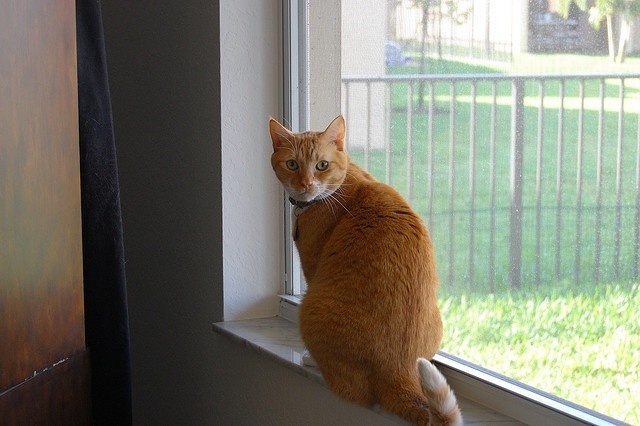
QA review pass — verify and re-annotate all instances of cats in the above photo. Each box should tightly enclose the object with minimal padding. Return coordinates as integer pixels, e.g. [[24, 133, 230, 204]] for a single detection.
[[268, 114, 463, 426]]
